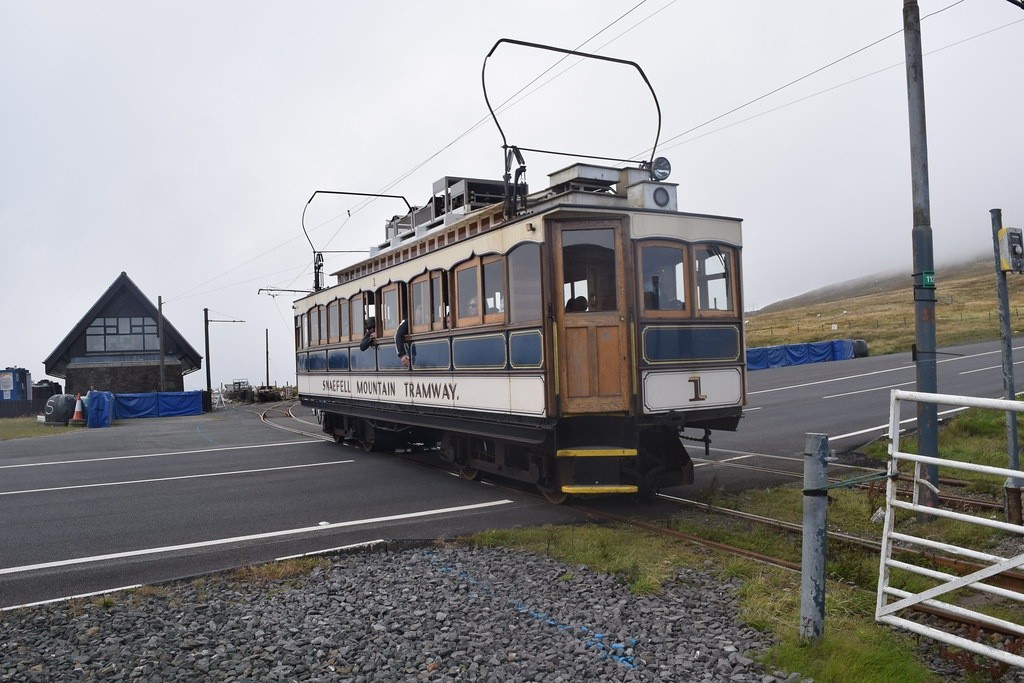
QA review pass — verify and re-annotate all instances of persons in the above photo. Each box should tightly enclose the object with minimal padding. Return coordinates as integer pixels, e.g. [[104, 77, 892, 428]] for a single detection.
[[358, 291, 684, 367]]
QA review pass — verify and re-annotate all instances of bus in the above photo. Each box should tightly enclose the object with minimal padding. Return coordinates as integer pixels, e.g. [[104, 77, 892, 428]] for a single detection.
[[254, 38, 750, 505]]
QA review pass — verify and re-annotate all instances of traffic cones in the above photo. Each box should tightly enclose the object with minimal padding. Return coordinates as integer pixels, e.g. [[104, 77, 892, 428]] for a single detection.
[[72, 392, 85, 420]]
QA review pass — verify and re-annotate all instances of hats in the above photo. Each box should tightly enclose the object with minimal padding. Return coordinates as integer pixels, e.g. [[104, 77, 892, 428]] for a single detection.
[[366, 317, 375, 328]]
